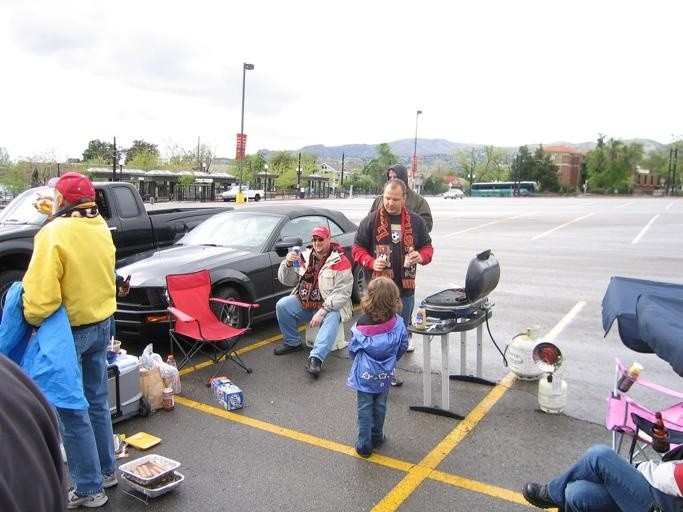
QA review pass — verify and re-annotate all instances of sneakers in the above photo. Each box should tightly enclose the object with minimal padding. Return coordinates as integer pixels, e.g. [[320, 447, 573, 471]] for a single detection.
[[370, 432, 387, 449], [65, 485, 111, 510], [355, 440, 372, 459], [100, 469, 119, 489], [273, 340, 305, 356], [521, 481, 566, 510], [305, 354, 323, 377]]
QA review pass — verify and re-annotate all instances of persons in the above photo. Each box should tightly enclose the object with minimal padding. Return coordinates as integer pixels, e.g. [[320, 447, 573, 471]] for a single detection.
[[21, 171, 117, 509], [351, 177, 434, 387], [369, 163, 434, 352], [522, 444, 683, 512], [274, 226, 354, 376], [348, 275, 408, 459]]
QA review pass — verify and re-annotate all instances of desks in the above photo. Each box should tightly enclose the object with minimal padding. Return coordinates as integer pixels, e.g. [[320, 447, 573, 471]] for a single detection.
[[407, 308, 499, 422]]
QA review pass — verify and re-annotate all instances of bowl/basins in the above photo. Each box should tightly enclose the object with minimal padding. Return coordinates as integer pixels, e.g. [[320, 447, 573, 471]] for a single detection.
[[118, 453, 182, 486], [105, 339, 121, 353], [120, 471, 185, 498]]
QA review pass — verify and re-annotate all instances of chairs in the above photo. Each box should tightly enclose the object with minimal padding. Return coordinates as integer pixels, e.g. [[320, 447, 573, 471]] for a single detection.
[[158, 269, 258, 388], [601, 355, 682, 472]]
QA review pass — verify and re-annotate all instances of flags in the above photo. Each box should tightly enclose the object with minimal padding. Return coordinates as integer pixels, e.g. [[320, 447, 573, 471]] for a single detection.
[[244, 64, 254, 70], [417, 111, 423, 114]]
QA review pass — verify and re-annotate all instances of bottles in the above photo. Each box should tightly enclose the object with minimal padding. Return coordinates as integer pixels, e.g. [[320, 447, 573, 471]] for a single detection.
[[651, 411, 670, 452], [165, 355, 177, 369], [117, 274, 133, 298], [617, 361, 644, 393], [160, 377, 175, 411], [404, 247, 416, 270], [376, 248, 387, 263], [415, 304, 427, 330], [31, 198, 55, 215]]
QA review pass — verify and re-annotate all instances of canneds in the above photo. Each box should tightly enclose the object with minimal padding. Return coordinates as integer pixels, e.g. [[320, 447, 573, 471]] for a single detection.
[[162, 388, 175, 411], [291, 246, 301, 268]]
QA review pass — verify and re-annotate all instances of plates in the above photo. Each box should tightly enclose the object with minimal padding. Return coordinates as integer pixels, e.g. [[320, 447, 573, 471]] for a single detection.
[[124, 432, 162, 449]]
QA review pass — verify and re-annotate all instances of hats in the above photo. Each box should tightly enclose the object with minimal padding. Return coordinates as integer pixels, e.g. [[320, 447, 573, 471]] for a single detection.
[[48, 170, 97, 209], [310, 224, 331, 239]]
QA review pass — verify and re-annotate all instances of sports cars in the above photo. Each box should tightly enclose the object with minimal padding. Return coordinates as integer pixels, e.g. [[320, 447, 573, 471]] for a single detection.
[[112, 204, 365, 351]]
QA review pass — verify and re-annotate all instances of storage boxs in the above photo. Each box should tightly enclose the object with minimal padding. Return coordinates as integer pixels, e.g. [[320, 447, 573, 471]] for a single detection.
[[101, 349, 149, 427]]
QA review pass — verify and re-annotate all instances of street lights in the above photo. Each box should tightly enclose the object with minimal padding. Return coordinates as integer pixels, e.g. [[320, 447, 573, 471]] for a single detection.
[[345, 173, 347, 180], [239, 63, 253, 194], [337, 152, 347, 185], [202, 163, 205, 173], [296, 152, 302, 191], [264, 164, 267, 201], [55, 156, 60, 178], [467, 167, 475, 197], [412, 110, 422, 192], [118, 159, 123, 182], [3, 186, 6, 202]]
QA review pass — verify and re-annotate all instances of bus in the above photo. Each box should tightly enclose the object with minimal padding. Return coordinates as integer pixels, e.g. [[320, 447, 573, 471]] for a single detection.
[[471, 181, 537, 197]]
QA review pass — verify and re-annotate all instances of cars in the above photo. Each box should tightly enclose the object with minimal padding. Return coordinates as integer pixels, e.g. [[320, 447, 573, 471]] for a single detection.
[[442, 188, 463, 199]]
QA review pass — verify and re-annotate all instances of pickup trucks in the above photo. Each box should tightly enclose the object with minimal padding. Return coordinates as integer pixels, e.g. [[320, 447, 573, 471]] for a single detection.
[[0, 181, 234, 326], [220, 185, 263, 202]]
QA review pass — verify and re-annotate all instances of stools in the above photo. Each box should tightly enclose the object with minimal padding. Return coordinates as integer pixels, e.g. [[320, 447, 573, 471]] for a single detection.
[[303, 317, 347, 352]]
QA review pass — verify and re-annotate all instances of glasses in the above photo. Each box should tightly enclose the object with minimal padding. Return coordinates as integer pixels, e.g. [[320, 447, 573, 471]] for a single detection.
[[311, 237, 325, 243]]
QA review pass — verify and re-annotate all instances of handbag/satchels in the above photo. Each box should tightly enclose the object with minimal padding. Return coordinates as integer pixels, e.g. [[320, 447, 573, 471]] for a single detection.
[[138, 363, 168, 413]]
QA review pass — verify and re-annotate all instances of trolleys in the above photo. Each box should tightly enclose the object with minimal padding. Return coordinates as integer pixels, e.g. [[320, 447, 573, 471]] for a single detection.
[[104, 353, 152, 427]]
